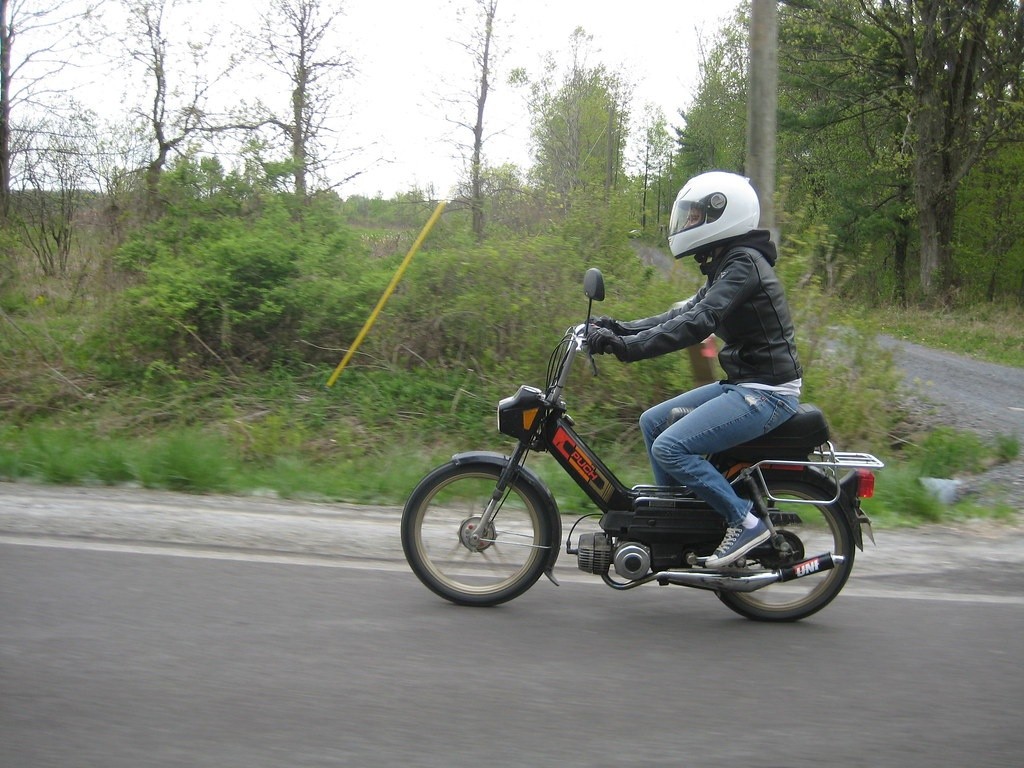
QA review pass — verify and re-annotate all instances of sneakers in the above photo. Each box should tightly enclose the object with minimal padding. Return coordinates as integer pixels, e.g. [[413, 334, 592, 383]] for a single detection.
[[705, 517, 771, 569]]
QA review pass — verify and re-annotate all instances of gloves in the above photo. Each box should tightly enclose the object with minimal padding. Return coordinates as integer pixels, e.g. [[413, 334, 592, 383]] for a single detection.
[[588, 327, 625, 357], [595, 317, 616, 330]]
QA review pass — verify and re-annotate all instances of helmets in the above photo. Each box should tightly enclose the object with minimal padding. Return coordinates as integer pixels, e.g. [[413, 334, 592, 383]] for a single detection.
[[667, 171, 760, 259]]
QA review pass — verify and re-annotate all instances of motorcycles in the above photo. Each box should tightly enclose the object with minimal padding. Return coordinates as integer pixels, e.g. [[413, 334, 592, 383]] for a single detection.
[[400, 268, 886, 627]]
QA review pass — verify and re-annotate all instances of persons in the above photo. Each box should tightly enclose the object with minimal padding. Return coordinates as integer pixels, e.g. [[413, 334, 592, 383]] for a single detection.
[[583, 172, 803, 569]]
